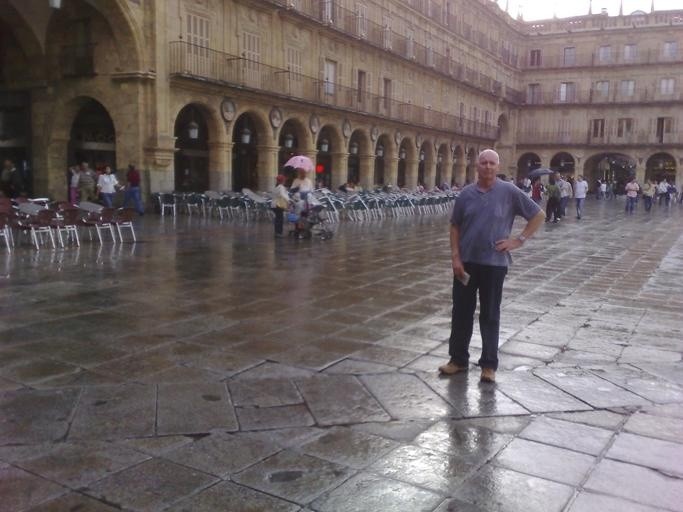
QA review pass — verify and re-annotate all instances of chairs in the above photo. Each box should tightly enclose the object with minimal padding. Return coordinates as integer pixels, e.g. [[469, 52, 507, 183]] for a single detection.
[[1, 188, 138, 255]]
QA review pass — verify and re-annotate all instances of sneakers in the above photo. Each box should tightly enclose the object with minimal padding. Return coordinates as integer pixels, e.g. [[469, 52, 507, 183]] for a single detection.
[[556, 217, 561, 221], [481, 365, 497, 382], [439, 361, 470, 377]]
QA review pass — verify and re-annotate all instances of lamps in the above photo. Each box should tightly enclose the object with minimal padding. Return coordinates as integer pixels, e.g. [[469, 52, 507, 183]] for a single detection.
[[319, 138, 329, 153], [350, 141, 360, 154], [284, 128, 294, 148], [239, 118, 251, 144], [376, 144, 384, 157], [187, 109, 200, 139], [400, 147, 407, 160]]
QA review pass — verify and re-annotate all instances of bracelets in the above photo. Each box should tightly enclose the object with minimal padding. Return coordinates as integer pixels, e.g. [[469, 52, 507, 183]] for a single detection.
[[518, 234, 525, 242]]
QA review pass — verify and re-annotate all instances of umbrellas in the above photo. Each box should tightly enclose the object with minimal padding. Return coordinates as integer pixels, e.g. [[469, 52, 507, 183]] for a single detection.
[[527, 168, 554, 176], [283, 155, 315, 172]]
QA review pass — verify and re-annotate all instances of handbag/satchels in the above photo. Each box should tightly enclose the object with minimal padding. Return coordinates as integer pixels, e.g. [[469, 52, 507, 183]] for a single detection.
[[288, 211, 300, 223]]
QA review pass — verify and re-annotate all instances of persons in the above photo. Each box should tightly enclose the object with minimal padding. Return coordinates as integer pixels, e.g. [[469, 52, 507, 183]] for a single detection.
[[508, 174, 676, 224], [289, 171, 313, 198], [438, 149, 546, 382], [117, 163, 145, 216], [270, 175, 294, 239], [337, 180, 362, 193], [95, 165, 127, 211], [0, 155, 98, 204], [416, 182, 466, 195]]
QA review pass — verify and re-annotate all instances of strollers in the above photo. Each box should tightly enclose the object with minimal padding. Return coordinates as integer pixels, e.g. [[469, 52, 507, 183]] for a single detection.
[[286, 191, 334, 241]]
